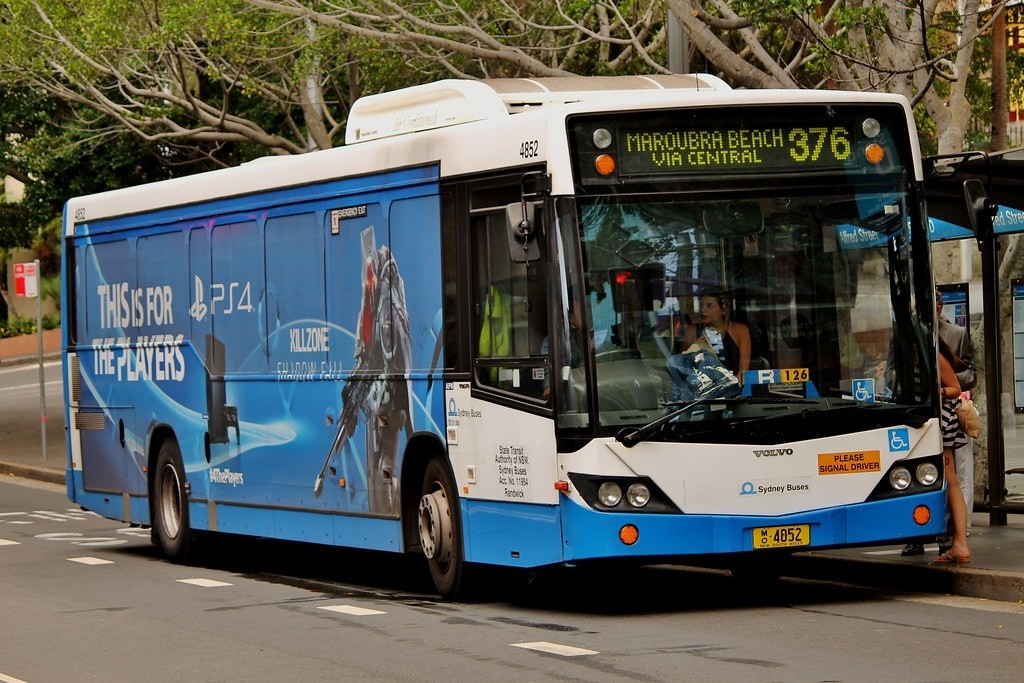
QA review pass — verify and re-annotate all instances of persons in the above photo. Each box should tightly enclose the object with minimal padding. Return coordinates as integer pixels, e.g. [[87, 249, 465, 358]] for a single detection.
[[679, 292, 751, 387], [659, 304, 703, 346], [540, 284, 601, 399], [882, 288, 976, 563]]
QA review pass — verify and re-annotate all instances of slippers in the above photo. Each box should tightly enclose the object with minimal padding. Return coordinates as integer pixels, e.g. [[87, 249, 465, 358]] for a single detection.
[[934, 550, 971, 563]]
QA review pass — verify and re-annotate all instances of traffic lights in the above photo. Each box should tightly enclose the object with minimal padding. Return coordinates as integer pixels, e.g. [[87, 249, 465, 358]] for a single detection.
[[607, 266, 640, 313]]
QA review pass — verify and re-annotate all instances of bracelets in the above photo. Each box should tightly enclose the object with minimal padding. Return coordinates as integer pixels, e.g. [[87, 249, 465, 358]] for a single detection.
[[942, 388, 946, 395]]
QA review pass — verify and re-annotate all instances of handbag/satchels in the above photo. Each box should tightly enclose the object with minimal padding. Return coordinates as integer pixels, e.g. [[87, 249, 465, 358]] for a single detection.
[[956, 397, 981, 439]]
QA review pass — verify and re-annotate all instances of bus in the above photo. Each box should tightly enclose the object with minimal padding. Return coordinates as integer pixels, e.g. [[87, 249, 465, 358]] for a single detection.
[[59, 72, 1000, 604]]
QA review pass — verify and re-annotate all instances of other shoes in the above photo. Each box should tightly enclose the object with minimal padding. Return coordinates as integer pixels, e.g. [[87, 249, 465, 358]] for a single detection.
[[938, 544, 952, 556], [901, 543, 924, 555]]
